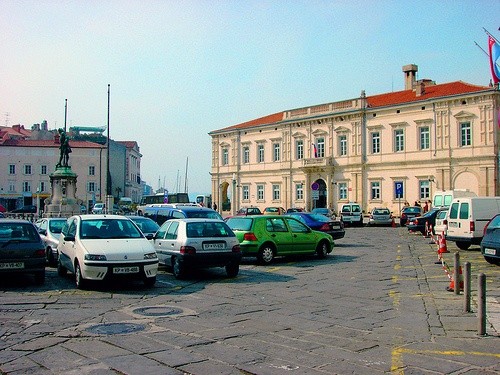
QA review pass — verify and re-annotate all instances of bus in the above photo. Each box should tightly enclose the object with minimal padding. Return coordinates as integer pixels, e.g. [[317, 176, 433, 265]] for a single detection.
[[195, 194, 230, 208], [136, 192, 189, 217]]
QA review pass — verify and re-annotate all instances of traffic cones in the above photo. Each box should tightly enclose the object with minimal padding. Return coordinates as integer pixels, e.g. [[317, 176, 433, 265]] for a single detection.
[[425, 221, 451, 264], [392, 219, 396, 228], [446, 266, 464, 293]]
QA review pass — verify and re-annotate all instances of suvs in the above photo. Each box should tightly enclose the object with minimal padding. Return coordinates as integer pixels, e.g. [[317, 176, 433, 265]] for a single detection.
[[311, 208, 333, 216], [91, 203, 105, 214], [223, 207, 262, 223], [143, 203, 225, 227]]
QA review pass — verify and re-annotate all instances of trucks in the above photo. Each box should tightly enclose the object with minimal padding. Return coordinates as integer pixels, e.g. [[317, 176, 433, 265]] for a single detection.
[[432, 190, 478, 209], [446, 197, 500, 249], [339, 204, 365, 225]]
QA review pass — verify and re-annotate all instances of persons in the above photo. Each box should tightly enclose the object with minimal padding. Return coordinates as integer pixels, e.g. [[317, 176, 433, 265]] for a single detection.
[[415, 201, 421, 206], [56, 128, 71, 166], [428, 200, 432, 211], [213, 202, 217, 211], [423, 201, 428, 213], [400, 202, 410, 212]]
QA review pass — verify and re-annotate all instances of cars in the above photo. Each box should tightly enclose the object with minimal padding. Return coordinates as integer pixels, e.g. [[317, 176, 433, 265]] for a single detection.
[[80, 206, 87, 213], [368, 207, 396, 227], [262, 206, 286, 215], [434, 210, 448, 236], [286, 208, 304, 214], [0, 218, 48, 283], [405, 208, 450, 235], [480, 214, 500, 266], [146, 218, 241, 279], [280, 212, 345, 241], [397, 206, 422, 226], [125, 216, 160, 240], [32, 217, 69, 266], [57, 213, 160, 286], [225, 214, 335, 264]]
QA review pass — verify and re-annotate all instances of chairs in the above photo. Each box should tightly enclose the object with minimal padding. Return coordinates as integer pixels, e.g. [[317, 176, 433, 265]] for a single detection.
[[86, 226, 119, 236], [11, 230, 22, 237], [375, 211, 378, 213], [384, 211, 387, 213], [188, 229, 215, 237]]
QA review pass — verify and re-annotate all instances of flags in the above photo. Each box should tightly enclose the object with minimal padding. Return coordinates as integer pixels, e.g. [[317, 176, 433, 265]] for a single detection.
[[489, 36, 500, 84], [314, 145, 318, 158]]
